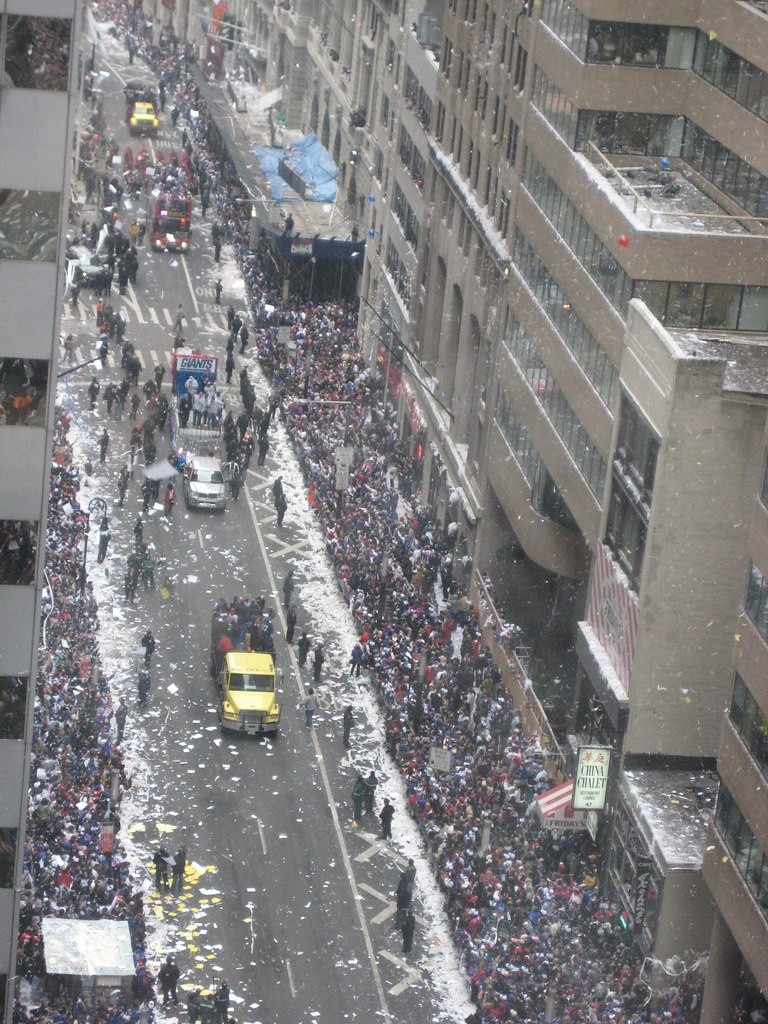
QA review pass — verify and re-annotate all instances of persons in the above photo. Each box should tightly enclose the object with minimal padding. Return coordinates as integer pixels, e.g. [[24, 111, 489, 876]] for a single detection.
[[172, 303, 186, 332], [313, 643, 324, 680], [215, 279, 223, 304], [142, 631, 156, 669], [0, 0, 236, 1024], [158, 956, 181, 1006], [236, 193, 768, 1024], [283, 571, 295, 608], [300, 688, 320, 727], [297, 633, 311, 668], [285, 213, 295, 230], [379, 798, 395, 840], [401, 909, 415, 952], [135, 666, 151, 708], [98, 429, 109, 462], [342, 706, 355, 748], [286, 605, 297, 644]]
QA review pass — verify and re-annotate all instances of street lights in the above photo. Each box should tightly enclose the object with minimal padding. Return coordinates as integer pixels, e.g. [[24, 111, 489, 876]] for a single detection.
[[78, 498, 109, 614], [298, 399, 351, 520], [235, 198, 276, 291]]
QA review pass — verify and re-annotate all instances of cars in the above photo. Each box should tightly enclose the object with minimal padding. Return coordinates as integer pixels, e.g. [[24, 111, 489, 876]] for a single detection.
[[183, 456, 226, 512], [64, 246, 107, 286]]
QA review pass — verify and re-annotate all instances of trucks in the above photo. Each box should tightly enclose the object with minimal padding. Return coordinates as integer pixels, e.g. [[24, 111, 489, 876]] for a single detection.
[[167, 353, 223, 454], [212, 642, 280, 738], [126, 101, 159, 136]]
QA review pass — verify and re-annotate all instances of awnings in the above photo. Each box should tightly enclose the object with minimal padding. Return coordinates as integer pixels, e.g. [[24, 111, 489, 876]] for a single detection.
[[535, 779, 592, 830]]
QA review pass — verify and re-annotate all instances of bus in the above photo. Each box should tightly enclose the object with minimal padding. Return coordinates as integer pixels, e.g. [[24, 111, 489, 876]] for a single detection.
[[151, 193, 192, 252]]
